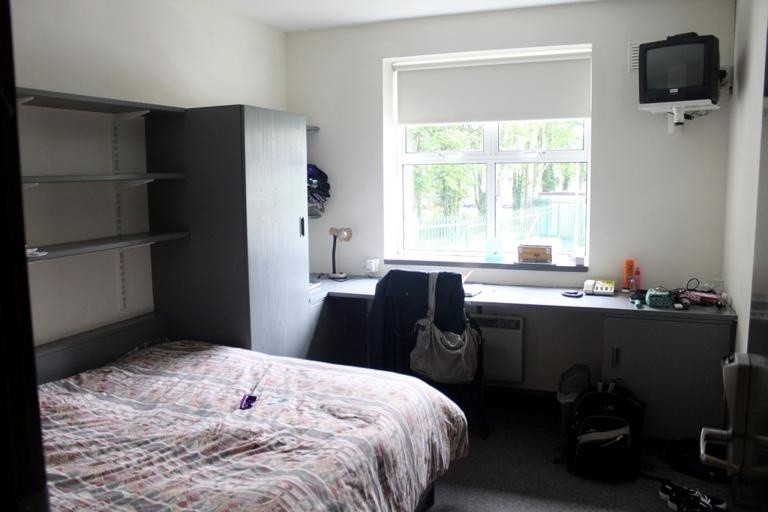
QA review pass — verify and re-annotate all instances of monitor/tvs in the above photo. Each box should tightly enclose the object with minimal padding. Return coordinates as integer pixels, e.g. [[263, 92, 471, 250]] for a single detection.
[[639, 35, 720, 104]]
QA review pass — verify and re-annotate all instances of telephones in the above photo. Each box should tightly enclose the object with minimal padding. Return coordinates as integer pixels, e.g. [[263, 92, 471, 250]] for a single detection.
[[583, 279, 616, 295]]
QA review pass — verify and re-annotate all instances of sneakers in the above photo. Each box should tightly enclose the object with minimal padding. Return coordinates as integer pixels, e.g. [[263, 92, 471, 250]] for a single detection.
[[659, 479, 728, 511]]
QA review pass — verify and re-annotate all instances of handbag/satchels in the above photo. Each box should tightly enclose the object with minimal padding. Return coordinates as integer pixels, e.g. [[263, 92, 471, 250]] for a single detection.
[[410, 271, 480, 383]]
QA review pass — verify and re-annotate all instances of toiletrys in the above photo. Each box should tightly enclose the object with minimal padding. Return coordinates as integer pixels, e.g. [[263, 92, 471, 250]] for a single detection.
[[634, 266, 641, 289], [623, 257, 633, 290]]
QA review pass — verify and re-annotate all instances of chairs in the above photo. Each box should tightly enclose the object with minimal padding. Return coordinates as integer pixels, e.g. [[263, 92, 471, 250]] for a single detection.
[[372, 268, 485, 390]]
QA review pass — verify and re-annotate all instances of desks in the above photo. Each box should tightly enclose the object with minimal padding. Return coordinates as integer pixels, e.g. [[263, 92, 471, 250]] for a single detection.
[[301, 271, 741, 451]]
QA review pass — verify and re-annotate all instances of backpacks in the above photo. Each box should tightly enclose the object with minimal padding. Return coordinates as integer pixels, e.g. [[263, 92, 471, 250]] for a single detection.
[[556, 380, 644, 486]]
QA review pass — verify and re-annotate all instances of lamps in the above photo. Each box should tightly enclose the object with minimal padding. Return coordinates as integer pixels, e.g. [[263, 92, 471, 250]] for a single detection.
[[329, 227, 352, 278]]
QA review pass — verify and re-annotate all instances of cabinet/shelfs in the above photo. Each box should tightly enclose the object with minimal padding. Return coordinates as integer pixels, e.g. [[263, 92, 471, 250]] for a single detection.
[[144, 106, 308, 370], [14, 85, 189, 261]]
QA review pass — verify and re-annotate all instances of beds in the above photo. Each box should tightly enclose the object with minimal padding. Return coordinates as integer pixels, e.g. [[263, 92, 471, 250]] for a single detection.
[[36, 309, 436, 510]]
